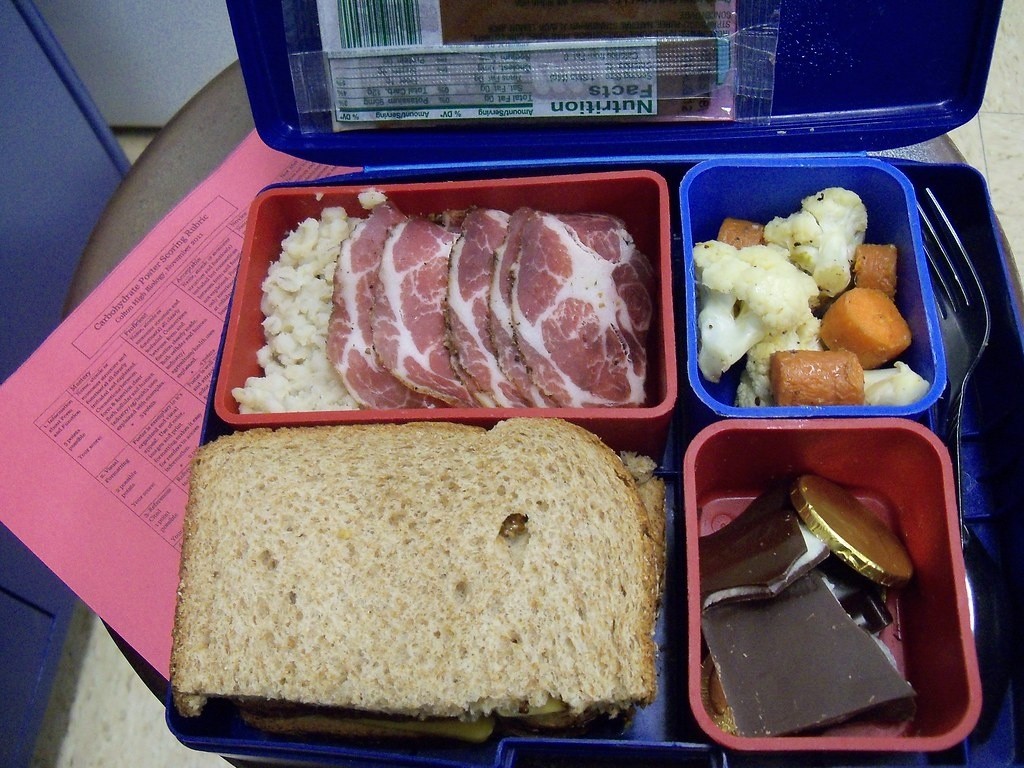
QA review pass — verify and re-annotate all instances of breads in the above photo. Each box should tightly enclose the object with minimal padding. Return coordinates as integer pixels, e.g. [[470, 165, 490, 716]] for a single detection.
[[169, 416, 667, 740]]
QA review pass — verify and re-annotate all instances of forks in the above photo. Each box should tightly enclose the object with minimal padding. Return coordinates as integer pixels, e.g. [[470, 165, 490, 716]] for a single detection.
[[914, 185, 994, 637]]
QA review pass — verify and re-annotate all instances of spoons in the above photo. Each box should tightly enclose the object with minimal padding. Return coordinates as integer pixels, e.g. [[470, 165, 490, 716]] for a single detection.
[[946, 403, 1012, 749]]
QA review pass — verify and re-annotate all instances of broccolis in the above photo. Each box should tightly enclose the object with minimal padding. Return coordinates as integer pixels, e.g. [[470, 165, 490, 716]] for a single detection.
[[691, 186, 932, 409]]
